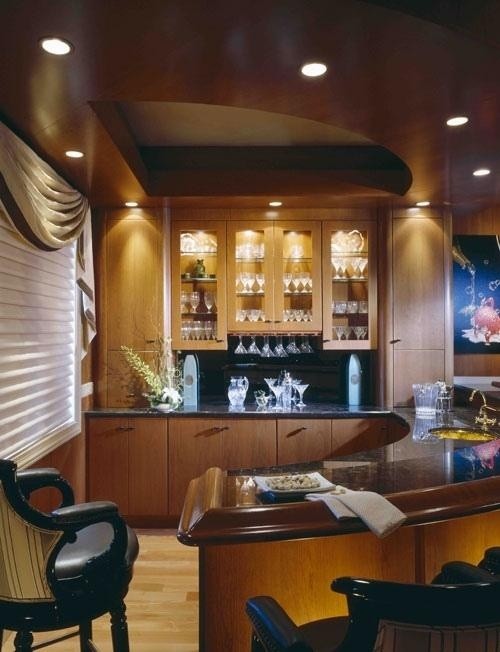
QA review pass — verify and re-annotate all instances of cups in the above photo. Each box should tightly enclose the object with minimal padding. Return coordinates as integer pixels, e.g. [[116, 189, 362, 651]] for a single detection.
[[236, 244, 264, 262], [236, 308, 312, 322], [332, 299, 368, 314], [181, 273, 216, 278], [413, 414, 437, 443], [413, 383, 438, 415]]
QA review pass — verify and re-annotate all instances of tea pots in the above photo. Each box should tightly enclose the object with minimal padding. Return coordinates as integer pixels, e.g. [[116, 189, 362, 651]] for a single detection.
[[291, 242, 304, 262], [227, 375, 249, 405]]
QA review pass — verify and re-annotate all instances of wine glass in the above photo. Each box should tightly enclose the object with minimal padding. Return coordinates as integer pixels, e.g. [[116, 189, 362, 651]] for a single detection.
[[235, 333, 312, 358], [253, 370, 309, 408], [180, 291, 217, 313], [332, 326, 368, 340], [181, 320, 216, 340], [236, 272, 312, 294], [333, 257, 368, 278]]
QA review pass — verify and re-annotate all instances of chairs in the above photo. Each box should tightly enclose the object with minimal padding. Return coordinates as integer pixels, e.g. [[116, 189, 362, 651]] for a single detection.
[[240, 559, 500, 652], [477, 547, 499, 577], [0, 457, 142, 651]]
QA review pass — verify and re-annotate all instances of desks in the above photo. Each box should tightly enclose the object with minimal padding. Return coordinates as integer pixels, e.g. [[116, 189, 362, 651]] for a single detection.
[[453, 373, 500, 407]]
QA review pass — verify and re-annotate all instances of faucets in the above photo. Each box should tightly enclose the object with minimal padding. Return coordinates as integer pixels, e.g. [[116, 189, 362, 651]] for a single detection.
[[469, 388, 486, 406]]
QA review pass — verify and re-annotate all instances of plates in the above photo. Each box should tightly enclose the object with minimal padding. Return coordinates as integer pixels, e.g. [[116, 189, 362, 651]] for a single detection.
[[254, 473, 338, 494]]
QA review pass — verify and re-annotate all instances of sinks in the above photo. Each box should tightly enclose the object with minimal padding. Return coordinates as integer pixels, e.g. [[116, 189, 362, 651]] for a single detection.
[[428, 427, 500, 440]]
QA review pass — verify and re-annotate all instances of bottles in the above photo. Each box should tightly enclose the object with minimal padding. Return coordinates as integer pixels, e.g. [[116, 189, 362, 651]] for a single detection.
[[194, 258, 205, 278], [451, 236, 470, 271]]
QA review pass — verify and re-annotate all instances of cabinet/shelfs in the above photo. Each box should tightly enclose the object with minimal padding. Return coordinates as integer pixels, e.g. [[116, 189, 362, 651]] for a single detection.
[[172, 218, 225, 354], [384, 211, 452, 409], [273, 413, 332, 466], [163, 411, 276, 527], [229, 218, 324, 335], [82, 409, 168, 528], [321, 219, 377, 353], [325, 417, 387, 464], [92, 210, 172, 405]]
